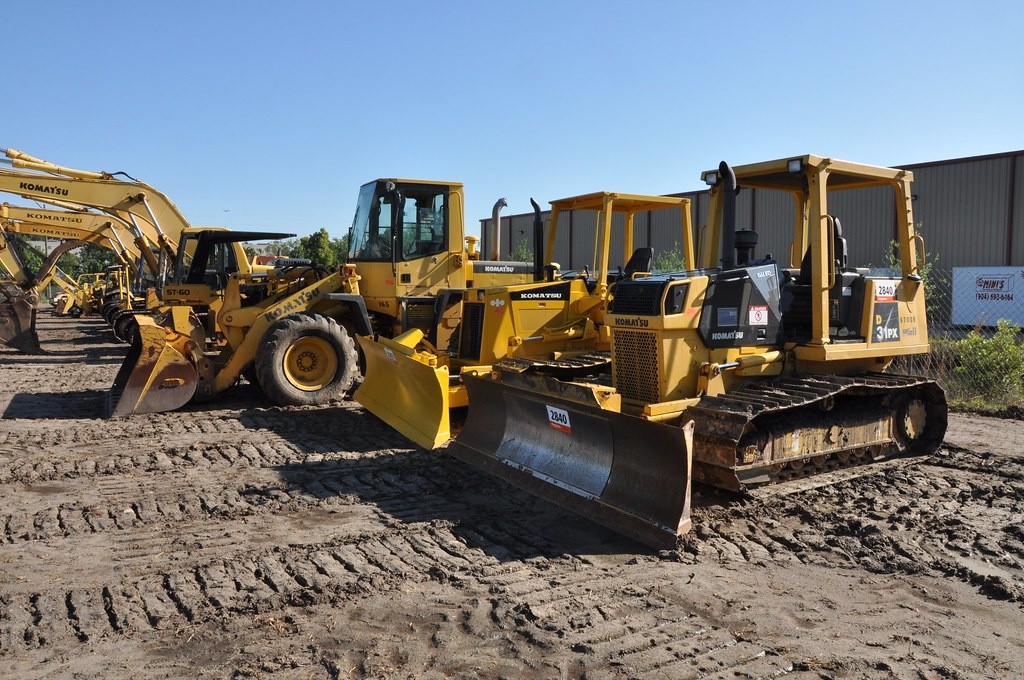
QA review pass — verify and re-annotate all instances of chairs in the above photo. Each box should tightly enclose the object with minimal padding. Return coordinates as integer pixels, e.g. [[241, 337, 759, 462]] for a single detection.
[[615, 246, 655, 281], [801, 217, 848, 285]]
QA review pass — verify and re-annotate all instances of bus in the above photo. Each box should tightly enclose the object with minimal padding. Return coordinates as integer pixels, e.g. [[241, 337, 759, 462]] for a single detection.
[[445, 155, 950, 551]]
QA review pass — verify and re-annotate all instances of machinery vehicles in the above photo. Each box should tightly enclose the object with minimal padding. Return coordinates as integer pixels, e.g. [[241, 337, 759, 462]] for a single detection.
[[0, 138, 302, 345], [353, 190, 703, 452], [107, 168, 507, 413]]
